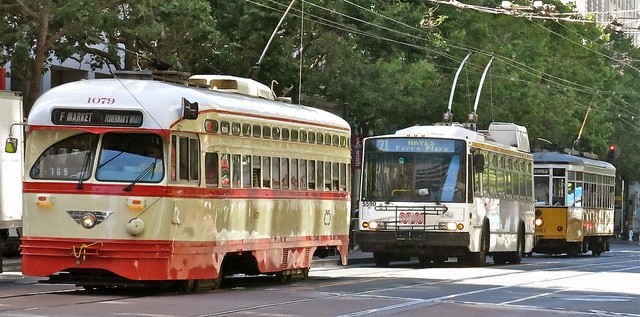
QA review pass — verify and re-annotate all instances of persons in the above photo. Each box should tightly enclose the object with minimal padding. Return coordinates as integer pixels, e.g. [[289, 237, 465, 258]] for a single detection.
[[567, 182, 574, 206]]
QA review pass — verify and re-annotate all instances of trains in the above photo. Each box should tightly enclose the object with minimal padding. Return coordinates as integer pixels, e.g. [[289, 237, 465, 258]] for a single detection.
[[4, 74, 352, 289], [531, 151, 615, 257]]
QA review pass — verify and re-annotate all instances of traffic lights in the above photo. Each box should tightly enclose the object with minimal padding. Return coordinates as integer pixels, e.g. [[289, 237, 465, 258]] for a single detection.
[[609, 145, 615, 158]]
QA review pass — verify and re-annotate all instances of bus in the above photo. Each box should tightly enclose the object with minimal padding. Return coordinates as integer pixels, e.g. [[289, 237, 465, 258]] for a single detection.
[[355, 121, 536, 267]]
